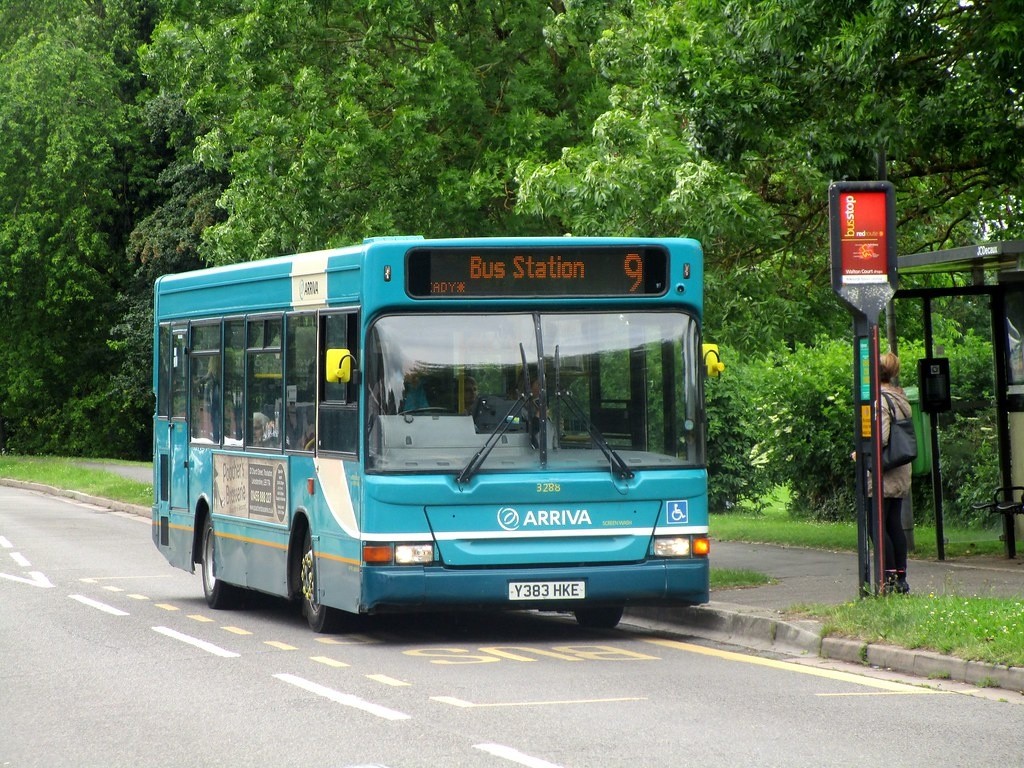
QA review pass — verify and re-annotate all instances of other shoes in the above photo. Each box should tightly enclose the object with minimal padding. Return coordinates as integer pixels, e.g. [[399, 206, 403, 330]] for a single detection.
[[865, 569, 910, 594]]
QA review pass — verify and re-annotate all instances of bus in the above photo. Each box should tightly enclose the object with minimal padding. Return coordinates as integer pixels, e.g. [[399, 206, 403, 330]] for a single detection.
[[151, 235, 726, 634]]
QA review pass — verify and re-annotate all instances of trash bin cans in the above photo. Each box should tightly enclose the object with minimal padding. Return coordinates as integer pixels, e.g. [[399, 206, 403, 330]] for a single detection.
[[901, 385, 933, 477]]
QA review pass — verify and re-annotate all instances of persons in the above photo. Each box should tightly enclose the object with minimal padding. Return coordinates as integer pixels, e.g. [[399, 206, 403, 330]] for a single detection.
[[851, 350, 918, 599], [195, 347, 244, 404], [251, 412, 276, 447], [512, 363, 560, 451], [367, 354, 430, 454], [452, 375, 481, 413]]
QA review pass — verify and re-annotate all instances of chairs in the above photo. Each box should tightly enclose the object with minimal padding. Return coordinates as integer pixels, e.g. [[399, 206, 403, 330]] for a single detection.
[[210, 373, 307, 449]]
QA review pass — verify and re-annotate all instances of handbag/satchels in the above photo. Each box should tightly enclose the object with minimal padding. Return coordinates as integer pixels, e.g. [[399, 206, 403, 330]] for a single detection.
[[860, 392, 918, 473]]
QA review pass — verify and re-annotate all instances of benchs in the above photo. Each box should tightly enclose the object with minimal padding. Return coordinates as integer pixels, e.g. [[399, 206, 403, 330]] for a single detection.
[[969, 485, 1024, 515]]
